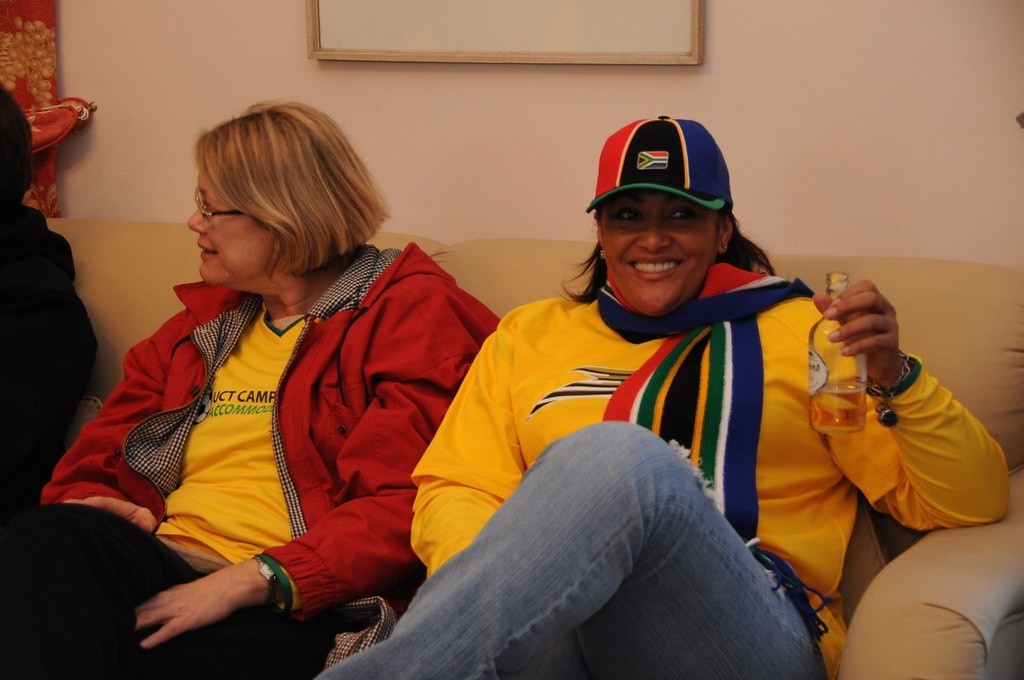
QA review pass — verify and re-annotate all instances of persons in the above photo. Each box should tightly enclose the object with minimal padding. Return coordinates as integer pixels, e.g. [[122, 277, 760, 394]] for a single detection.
[[3, 91, 506, 670], [317, 112, 1011, 680], [0, 94, 99, 498]]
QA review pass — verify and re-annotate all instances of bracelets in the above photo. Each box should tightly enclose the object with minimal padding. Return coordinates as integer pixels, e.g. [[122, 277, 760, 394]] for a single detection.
[[865, 349, 911, 427]]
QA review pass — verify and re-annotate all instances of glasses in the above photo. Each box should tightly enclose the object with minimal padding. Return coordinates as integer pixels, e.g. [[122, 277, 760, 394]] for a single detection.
[[195, 188, 243, 221]]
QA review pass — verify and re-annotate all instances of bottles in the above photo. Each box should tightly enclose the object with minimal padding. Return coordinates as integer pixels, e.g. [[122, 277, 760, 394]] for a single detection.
[[808, 274, 868, 433]]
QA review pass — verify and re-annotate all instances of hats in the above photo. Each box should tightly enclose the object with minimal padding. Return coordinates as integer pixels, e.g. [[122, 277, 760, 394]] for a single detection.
[[586, 116, 732, 213]]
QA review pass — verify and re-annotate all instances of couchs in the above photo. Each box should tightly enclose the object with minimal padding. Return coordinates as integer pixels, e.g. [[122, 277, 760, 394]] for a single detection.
[[47, 217, 1024, 680]]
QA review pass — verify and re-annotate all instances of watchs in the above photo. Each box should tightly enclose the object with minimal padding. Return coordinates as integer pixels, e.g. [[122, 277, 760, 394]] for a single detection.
[[254, 554, 279, 600]]
